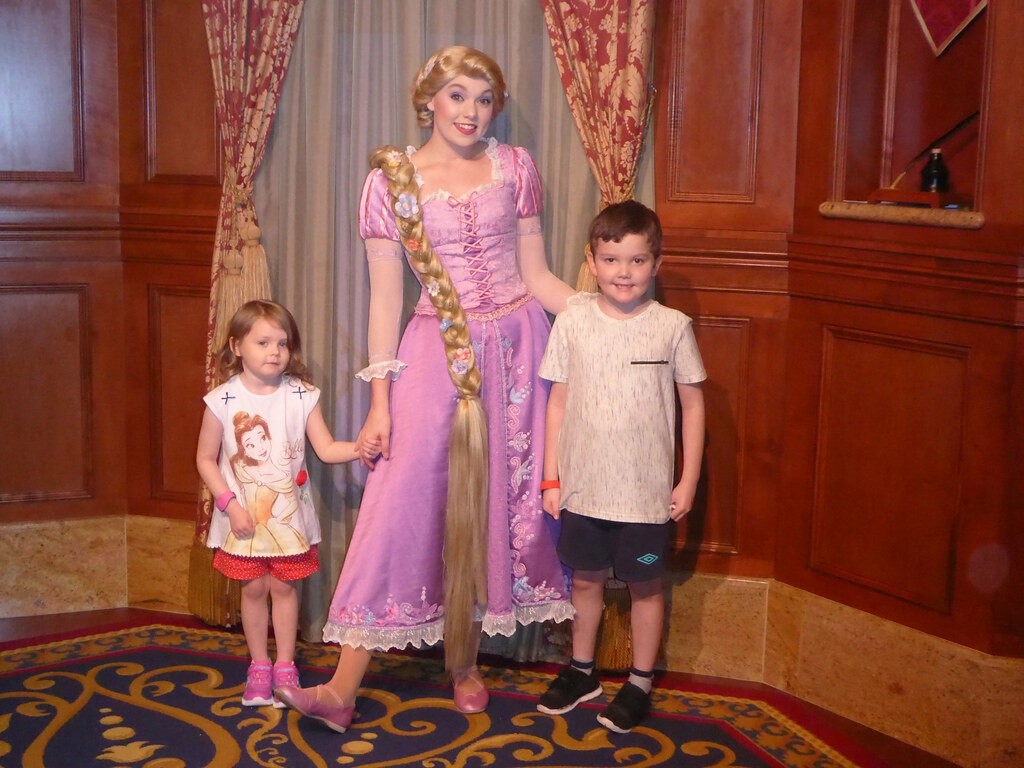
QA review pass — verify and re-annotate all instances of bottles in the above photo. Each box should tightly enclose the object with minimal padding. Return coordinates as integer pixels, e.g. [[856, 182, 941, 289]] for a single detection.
[[920, 149, 949, 193]]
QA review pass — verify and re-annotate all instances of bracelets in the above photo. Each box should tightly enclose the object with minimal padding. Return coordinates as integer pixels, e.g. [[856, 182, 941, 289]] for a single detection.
[[215, 492, 236, 512], [539, 480, 560, 490]]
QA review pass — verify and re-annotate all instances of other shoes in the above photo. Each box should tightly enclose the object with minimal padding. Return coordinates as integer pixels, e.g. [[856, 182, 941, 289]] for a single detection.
[[454, 665, 489, 714], [274, 684, 355, 733]]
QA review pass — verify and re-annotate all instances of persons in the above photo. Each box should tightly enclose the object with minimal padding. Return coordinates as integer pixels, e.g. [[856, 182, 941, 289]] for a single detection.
[[536, 199, 708, 735], [197, 299, 382, 707], [273, 45, 577, 730]]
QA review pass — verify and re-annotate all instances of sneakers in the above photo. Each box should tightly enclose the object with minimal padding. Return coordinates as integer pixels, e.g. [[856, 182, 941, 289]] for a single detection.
[[597, 681, 652, 733], [537, 666, 602, 715], [242, 660, 273, 706], [272, 661, 301, 708]]
